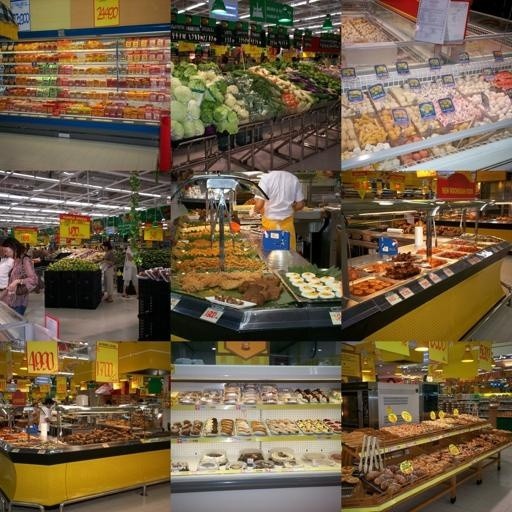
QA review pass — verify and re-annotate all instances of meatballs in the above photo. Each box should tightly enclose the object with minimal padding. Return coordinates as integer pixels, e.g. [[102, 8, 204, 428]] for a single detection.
[[341, 17, 389, 43], [341, 72, 512, 170]]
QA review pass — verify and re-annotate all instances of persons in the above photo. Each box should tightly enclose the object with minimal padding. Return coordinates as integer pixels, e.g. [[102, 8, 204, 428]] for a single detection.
[[4, 238, 37, 315], [99, 244, 107, 291], [0, 236, 14, 303], [94, 242, 115, 303], [255, 171, 304, 250], [121, 238, 139, 298], [39, 397, 55, 440]]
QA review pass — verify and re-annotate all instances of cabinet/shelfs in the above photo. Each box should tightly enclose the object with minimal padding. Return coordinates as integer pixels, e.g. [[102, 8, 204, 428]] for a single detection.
[[339, 393, 511, 511], [341, 195, 511, 341], [171, 376, 341, 478], [427, 200, 512, 258], [0, 399, 171, 510], [0, 22, 168, 146]]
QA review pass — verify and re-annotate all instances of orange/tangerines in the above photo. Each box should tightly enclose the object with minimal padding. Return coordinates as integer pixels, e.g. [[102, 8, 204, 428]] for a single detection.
[[45, 257, 100, 271]]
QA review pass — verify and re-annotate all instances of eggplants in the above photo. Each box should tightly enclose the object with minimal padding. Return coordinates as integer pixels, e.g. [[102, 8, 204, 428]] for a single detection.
[[288, 72, 331, 94]]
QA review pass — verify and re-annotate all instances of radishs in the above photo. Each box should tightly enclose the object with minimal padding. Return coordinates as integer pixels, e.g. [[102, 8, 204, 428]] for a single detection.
[[248, 65, 314, 113]]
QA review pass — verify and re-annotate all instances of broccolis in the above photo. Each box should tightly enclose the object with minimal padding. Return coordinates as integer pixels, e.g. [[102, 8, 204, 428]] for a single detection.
[[198, 71, 250, 119]]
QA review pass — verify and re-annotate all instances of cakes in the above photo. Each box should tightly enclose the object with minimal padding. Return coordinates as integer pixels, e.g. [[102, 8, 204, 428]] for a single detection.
[[170, 450, 341, 472], [169, 418, 342, 437]]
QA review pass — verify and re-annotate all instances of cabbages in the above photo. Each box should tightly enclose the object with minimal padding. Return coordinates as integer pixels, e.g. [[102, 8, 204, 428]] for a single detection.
[[171, 62, 238, 141]]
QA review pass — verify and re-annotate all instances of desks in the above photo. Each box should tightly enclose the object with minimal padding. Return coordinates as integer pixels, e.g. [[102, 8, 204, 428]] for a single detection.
[[135, 275, 171, 340]]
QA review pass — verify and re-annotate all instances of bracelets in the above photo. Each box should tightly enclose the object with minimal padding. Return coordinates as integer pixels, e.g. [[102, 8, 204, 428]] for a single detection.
[[21, 279, 24, 285]]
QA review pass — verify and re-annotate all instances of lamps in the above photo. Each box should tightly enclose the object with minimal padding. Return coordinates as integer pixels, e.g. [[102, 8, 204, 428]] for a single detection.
[[459, 343, 476, 364]]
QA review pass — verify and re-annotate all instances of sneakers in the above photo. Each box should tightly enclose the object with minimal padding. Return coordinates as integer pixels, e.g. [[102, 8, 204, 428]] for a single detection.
[[103, 296, 114, 305]]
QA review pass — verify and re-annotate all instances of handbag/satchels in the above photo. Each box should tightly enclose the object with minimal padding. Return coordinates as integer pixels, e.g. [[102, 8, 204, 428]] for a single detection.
[[16, 255, 44, 296]]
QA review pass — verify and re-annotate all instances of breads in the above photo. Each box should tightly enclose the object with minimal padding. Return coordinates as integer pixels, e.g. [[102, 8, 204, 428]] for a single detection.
[[170, 382, 342, 404], [342, 414, 508, 493]]
